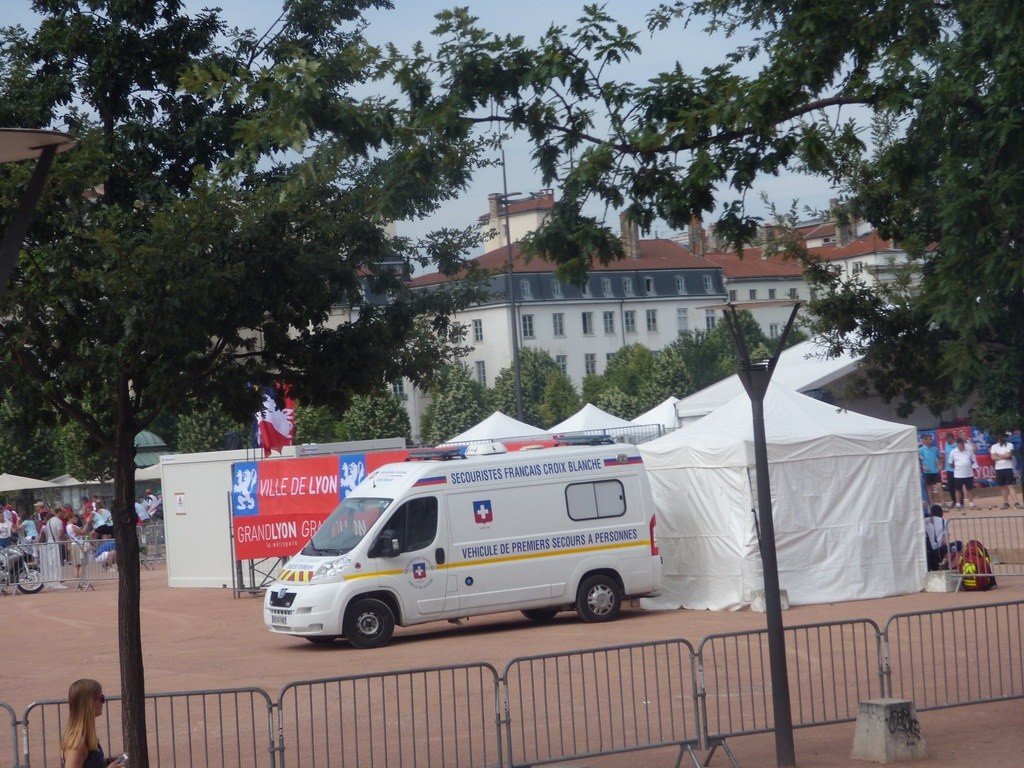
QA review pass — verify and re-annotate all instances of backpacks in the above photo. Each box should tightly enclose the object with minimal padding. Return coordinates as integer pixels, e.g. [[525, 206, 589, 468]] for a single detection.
[[958, 540, 997, 591]]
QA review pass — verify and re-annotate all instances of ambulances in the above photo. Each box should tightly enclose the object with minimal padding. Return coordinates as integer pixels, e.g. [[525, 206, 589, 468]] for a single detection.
[[262, 433, 667, 650]]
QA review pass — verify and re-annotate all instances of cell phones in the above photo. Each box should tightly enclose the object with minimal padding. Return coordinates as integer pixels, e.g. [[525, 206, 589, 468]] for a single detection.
[[117, 754, 129, 764]]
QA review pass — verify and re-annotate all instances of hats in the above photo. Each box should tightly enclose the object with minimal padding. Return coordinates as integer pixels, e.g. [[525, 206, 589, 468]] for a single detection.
[[33, 502, 44, 507], [145, 489, 152, 494], [80, 495, 88, 501], [62, 503, 71, 508], [6, 505, 12, 510]]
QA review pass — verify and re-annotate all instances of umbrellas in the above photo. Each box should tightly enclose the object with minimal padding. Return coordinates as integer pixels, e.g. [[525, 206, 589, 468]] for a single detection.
[[0, 462, 162, 506]]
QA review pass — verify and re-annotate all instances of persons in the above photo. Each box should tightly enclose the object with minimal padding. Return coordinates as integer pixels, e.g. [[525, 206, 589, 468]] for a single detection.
[[918, 407, 1024, 570], [0, 486, 163, 576], [60, 679, 127, 768]]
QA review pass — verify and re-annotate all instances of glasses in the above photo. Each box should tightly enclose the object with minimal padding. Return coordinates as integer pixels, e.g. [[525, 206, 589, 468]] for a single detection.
[[95, 695, 105, 703]]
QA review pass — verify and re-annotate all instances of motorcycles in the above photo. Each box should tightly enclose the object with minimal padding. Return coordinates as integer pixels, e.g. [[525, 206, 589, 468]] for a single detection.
[[0, 528, 45, 594]]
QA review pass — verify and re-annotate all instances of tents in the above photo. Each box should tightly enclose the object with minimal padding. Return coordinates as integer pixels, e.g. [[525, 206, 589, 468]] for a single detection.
[[434, 305, 1001, 611]]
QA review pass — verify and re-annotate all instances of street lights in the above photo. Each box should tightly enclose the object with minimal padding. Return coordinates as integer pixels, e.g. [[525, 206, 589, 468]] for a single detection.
[[498, 134, 526, 423], [691, 299, 817, 768]]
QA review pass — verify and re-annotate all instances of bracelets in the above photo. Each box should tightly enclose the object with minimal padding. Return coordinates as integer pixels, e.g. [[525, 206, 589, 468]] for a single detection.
[[106, 757, 110, 764]]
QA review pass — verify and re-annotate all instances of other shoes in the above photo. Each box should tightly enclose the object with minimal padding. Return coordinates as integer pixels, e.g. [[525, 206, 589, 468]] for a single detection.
[[942, 504, 948, 512], [970, 505, 981, 510], [1000, 503, 1009, 510], [53, 584, 68, 589], [105, 567, 116, 572], [953, 506, 960, 512], [949, 501, 955, 507], [1014, 503, 1024, 509], [937, 563, 949, 570]]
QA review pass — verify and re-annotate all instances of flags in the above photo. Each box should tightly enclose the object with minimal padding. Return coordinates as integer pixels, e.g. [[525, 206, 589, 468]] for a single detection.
[[252, 369, 296, 457]]
